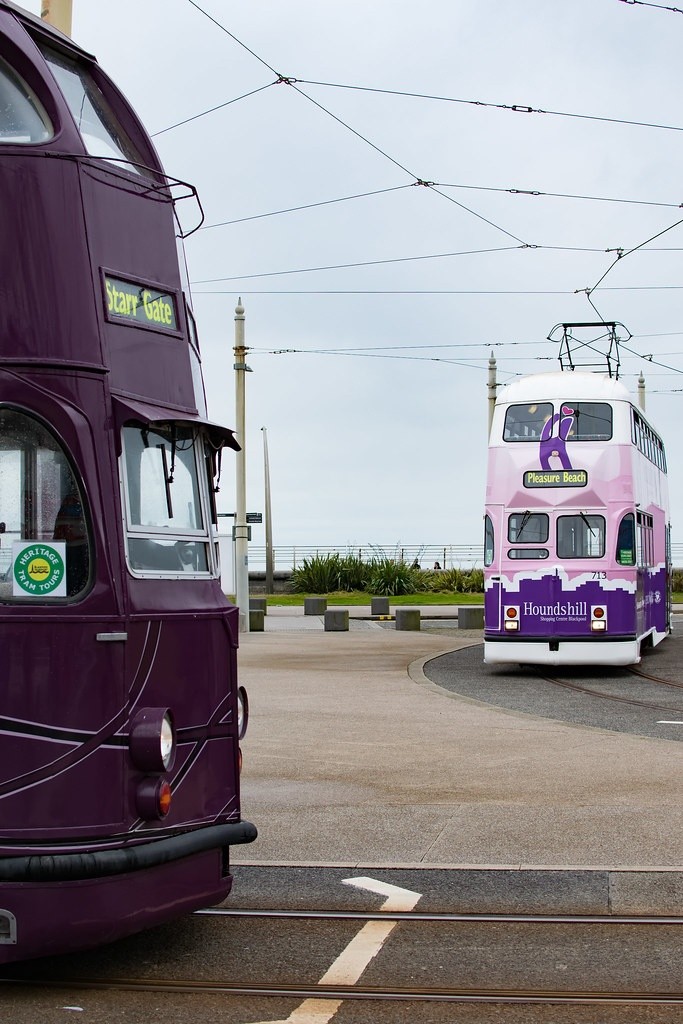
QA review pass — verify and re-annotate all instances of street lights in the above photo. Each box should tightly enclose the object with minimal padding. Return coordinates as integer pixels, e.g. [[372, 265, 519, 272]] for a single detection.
[[261, 426, 274, 595], [232, 362, 254, 633]]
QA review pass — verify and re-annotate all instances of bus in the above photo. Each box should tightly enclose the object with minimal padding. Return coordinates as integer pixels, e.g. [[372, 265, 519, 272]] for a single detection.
[[484, 321, 672, 666], [0, 0, 257, 966]]
